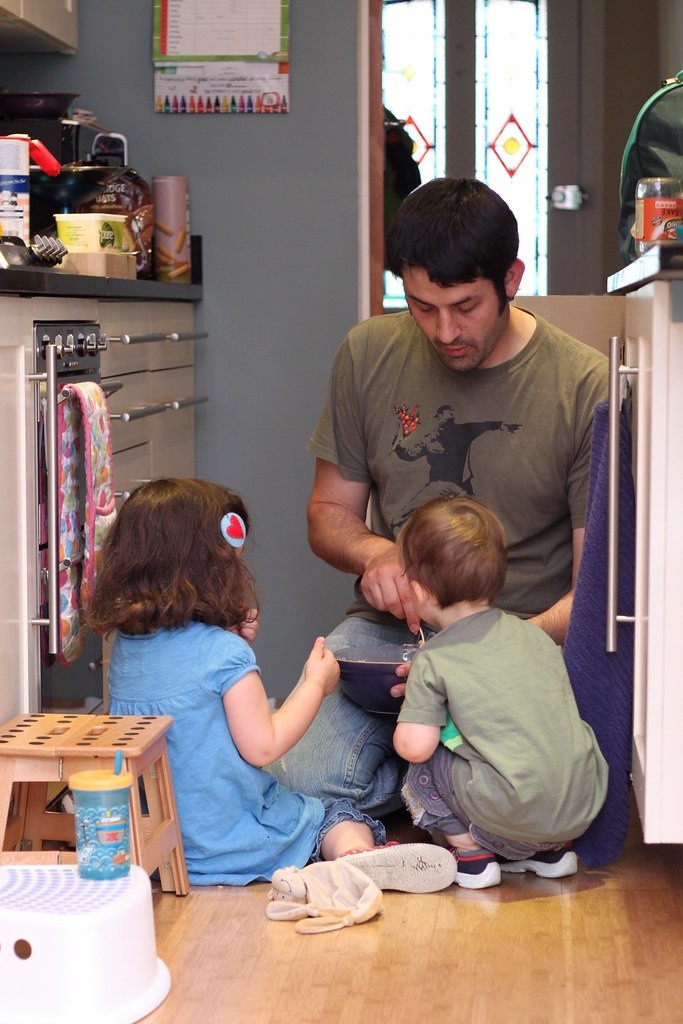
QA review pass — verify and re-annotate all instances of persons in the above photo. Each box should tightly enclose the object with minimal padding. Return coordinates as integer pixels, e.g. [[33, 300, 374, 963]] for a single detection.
[[85, 476, 459, 895], [261, 174, 619, 820], [393, 495, 611, 891]]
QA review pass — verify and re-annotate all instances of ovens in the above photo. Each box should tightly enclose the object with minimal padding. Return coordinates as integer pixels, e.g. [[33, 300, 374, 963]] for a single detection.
[[0, 291, 126, 720]]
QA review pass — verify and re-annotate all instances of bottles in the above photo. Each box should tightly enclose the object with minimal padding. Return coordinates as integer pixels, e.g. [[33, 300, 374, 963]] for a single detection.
[[634, 178, 682, 259]]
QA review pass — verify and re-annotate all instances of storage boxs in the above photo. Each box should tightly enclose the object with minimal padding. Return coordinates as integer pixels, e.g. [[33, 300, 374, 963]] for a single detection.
[[52, 212, 129, 253], [53, 251, 137, 280]]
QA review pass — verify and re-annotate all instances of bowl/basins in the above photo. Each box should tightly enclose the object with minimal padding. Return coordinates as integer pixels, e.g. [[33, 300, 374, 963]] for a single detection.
[[333, 643, 426, 714]]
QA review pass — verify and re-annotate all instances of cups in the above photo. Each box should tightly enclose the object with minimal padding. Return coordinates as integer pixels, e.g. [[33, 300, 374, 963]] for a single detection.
[[67, 768, 135, 880]]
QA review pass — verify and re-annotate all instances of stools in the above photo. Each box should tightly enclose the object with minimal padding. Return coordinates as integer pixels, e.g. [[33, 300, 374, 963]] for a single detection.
[[0, 712, 190, 898], [0, 863, 174, 1023]]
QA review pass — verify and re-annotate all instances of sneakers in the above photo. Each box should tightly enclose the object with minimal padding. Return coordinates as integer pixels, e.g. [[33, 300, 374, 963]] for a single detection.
[[492, 842, 578, 878], [451, 847, 501, 890]]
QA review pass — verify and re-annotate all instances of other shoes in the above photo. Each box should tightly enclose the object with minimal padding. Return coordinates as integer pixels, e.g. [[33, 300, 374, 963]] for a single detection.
[[332, 841, 458, 894]]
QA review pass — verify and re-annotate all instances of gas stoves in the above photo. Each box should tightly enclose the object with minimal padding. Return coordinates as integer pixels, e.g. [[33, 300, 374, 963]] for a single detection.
[[0, 234, 79, 275]]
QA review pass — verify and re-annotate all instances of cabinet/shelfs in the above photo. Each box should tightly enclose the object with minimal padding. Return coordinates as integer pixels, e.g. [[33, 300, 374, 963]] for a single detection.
[[0, 292, 211, 716], [609, 278, 682, 847]]
